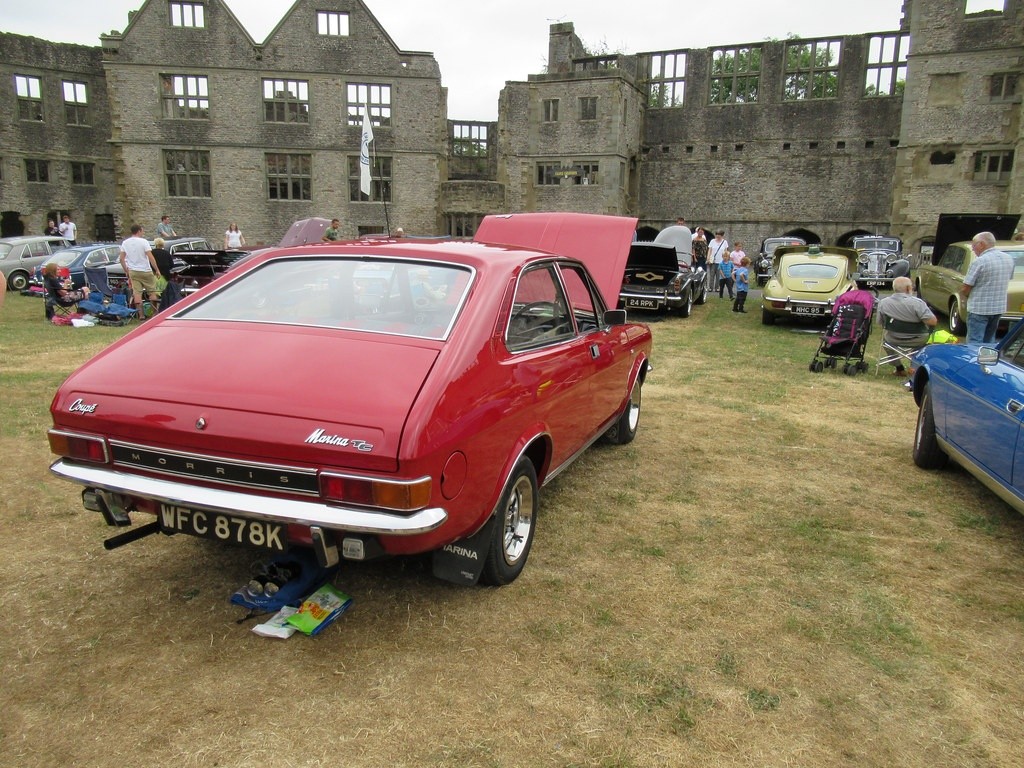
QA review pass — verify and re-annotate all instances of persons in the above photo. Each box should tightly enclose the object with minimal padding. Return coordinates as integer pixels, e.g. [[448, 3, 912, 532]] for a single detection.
[[224, 222, 246, 250], [44, 213, 77, 246], [876, 276, 939, 377], [676, 217, 751, 314], [157, 215, 177, 237], [120, 225, 174, 320], [960, 231, 1016, 343], [45, 264, 90, 306], [321, 219, 339, 241]]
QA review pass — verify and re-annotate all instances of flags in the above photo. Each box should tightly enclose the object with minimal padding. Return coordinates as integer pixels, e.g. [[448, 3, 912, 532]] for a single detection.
[[358, 105, 374, 195]]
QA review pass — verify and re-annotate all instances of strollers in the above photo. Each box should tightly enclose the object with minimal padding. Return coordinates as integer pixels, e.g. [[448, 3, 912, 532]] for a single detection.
[[808, 286, 882, 375]]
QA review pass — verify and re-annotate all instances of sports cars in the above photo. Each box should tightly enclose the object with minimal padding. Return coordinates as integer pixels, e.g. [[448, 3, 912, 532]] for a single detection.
[[616, 241, 709, 320]]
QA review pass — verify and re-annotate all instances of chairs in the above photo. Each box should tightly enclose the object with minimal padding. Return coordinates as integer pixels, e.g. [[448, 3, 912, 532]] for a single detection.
[[41, 266, 189, 327], [875, 314, 938, 378]]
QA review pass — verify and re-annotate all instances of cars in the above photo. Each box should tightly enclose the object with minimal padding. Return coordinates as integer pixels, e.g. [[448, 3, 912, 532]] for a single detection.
[[0, 235, 73, 293], [760, 244, 862, 326], [848, 233, 914, 290], [910, 239, 1024, 336], [40, 236, 251, 305], [906, 313, 1024, 517], [755, 237, 809, 286], [45, 211, 653, 586]]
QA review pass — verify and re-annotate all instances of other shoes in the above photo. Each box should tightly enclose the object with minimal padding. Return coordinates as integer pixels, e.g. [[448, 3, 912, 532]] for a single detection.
[[893, 370, 906, 376], [908, 368, 916, 374], [739, 309, 748, 313], [732, 308, 739, 312]]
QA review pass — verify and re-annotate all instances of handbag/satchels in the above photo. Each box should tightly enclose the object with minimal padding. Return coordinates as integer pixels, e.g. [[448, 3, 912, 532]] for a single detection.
[[705, 254, 715, 265]]
